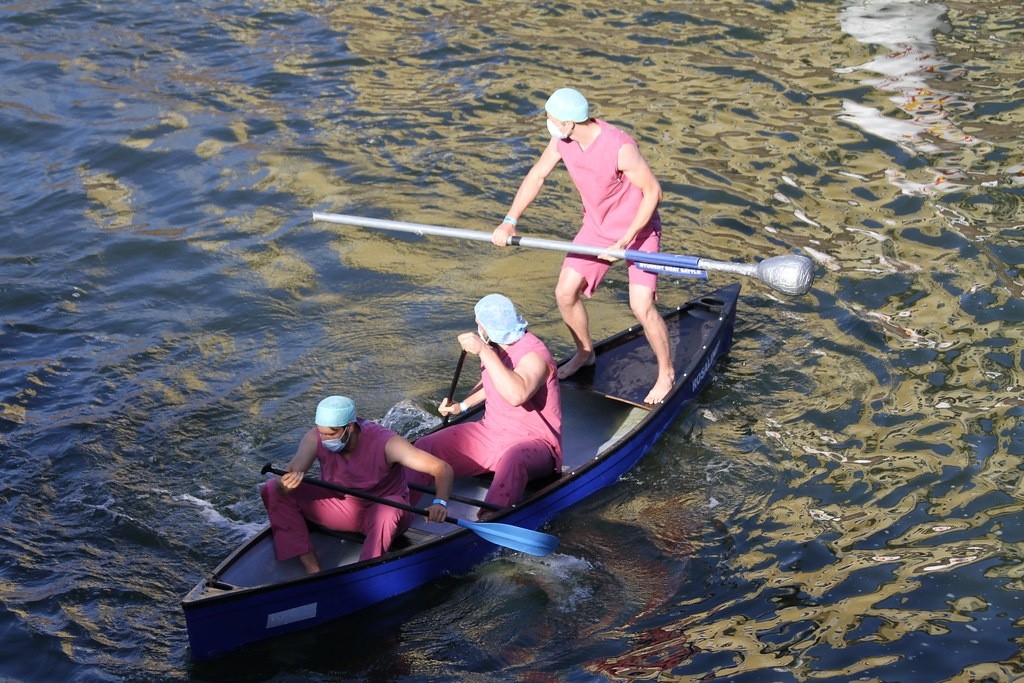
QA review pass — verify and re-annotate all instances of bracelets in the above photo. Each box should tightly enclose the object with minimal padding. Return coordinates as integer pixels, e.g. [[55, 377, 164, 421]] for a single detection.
[[459, 401, 468, 415], [504, 216, 517, 225], [433, 498, 448, 508]]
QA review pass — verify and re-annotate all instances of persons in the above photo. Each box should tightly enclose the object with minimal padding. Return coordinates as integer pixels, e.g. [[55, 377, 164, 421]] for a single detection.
[[260, 395, 455, 574], [491, 87, 677, 405], [405, 294, 563, 522]]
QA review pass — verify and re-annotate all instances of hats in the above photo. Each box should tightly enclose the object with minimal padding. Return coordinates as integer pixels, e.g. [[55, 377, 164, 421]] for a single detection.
[[545, 88, 589, 123], [315, 396, 357, 427], [474, 293, 528, 343]]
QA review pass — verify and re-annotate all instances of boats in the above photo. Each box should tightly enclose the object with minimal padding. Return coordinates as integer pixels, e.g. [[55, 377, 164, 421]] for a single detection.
[[181, 283, 744, 661]]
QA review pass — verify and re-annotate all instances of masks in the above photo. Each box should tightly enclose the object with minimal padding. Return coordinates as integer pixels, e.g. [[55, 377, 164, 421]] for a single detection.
[[322, 430, 349, 453], [477, 327, 489, 346], [547, 119, 568, 140]]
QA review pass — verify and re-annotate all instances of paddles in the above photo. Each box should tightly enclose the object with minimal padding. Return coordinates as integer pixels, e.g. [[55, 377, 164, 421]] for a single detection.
[[441, 349, 466, 427], [261, 461, 561, 557]]
